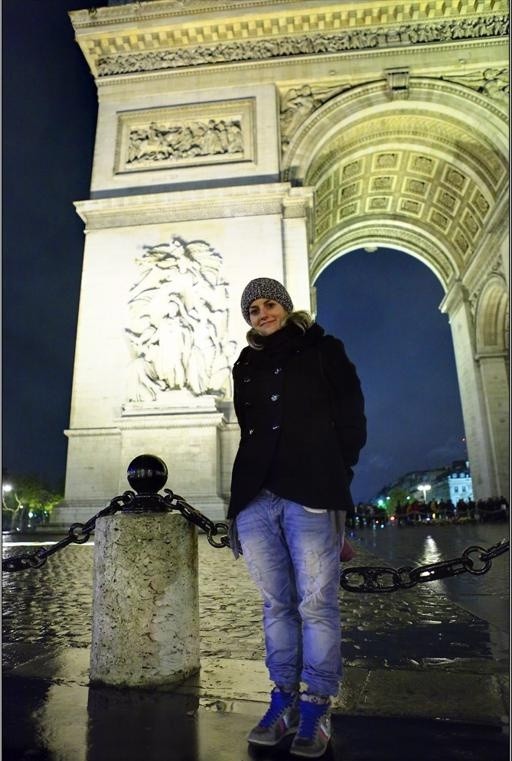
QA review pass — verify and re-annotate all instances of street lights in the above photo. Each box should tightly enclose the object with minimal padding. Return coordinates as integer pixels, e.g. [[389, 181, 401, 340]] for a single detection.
[[28, 512, 35, 529], [415, 485, 431, 503]]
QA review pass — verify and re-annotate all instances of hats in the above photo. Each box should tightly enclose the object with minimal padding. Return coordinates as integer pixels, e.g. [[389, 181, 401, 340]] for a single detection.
[[239, 277, 294, 327]]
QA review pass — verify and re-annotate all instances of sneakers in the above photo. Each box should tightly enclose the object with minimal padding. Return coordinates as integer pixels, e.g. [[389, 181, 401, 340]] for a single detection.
[[246, 686, 303, 747], [290, 691, 333, 760]]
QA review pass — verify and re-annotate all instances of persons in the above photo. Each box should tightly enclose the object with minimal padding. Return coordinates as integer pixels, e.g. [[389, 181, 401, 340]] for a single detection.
[[226, 277, 368, 759]]
